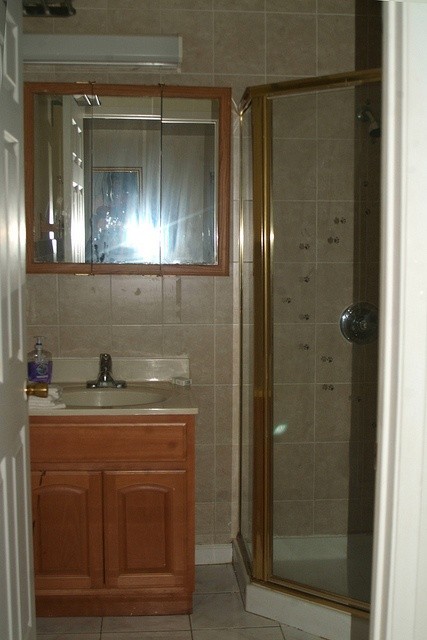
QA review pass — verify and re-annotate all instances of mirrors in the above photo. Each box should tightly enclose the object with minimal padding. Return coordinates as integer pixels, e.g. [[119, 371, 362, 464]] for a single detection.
[[22, 80, 232, 277]]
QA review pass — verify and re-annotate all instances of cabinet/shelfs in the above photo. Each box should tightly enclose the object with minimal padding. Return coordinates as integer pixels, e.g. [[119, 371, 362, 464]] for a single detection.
[[30, 413, 196, 615], [49, 100, 88, 262]]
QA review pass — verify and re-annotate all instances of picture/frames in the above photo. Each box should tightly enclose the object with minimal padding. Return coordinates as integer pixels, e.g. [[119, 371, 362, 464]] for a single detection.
[[88, 165, 144, 263]]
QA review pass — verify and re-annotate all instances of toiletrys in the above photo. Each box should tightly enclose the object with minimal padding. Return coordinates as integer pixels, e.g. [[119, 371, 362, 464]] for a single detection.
[[27, 336, 53, 384]]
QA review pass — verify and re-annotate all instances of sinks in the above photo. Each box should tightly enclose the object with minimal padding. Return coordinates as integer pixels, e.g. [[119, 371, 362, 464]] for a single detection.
[[62, 381, 168, 408]]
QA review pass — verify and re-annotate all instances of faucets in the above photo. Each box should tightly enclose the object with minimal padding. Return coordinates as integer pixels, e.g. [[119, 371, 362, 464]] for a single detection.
[[86, 353, 126, 389]]
